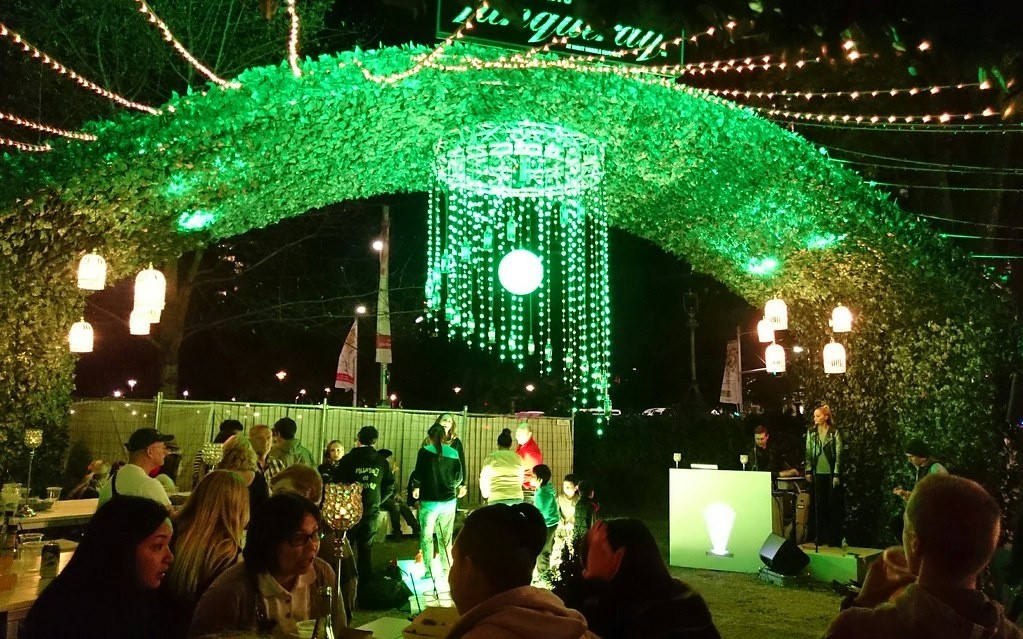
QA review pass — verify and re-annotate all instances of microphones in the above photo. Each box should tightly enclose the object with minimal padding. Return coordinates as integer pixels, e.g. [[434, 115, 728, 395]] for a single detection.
[[813, 422, 819, 429]]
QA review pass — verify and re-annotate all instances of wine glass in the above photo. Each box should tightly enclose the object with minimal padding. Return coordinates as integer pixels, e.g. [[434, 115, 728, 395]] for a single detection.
[[740, 455, 748, 470], [673, 453, 681, 468]]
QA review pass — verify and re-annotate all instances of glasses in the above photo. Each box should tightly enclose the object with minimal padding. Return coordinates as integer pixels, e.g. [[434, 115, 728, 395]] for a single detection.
[[282, 525, 326, 547]]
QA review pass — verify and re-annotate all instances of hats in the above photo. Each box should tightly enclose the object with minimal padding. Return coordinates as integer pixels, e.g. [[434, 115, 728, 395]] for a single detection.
[[125, 427, 174, 450], [906, 438, 926, 456]]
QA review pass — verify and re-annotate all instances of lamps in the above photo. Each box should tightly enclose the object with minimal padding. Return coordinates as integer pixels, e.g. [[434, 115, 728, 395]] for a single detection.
[[68, 193, 166, 353], [756, 287, 854, 375]]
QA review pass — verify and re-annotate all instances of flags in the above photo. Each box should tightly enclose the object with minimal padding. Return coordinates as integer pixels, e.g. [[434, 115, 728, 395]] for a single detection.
[[376, 224, 392, 363], [335, 323, 358, 391], [719, 340, 741, 404]]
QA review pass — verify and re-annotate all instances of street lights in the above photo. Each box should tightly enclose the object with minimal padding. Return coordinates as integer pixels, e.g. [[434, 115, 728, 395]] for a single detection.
[[680, 285, 703, 405], [736, 316, 787, 413]]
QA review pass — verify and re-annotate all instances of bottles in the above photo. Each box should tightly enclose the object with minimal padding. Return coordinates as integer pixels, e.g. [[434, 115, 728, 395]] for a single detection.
[[312, 585, 335, 639], [0, 510, 19, 593]]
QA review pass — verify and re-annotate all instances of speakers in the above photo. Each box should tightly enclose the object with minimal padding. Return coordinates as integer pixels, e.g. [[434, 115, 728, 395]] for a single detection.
[[759, 533, 811, 574]]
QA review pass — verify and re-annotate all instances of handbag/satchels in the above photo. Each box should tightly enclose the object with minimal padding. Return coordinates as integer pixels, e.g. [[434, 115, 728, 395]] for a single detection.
[[357, 566, 414, 609]]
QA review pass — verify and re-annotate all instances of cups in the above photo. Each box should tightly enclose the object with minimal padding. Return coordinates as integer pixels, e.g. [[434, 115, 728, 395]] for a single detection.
[[46, 486, 63, 512], [1, 482, 30, 516], [297, 619, 316, 639], [19, 533, 44, 573]]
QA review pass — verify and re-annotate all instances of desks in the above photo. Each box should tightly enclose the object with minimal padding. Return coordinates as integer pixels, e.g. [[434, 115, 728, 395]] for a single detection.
[[0, 495, 101, 639]]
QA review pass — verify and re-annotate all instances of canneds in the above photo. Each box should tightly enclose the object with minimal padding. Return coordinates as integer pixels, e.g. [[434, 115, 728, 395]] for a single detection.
[[40, 543, 60, 578]]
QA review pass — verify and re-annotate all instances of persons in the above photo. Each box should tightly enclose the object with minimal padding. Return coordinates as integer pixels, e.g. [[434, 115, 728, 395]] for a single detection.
[[804, 406, 848, 547], [20, 412, 600, 639], [557, 516, 722, 639], [819, 474, 1023, 639], [892, 439, 948, 502], [735, 424, 799, 478]]
